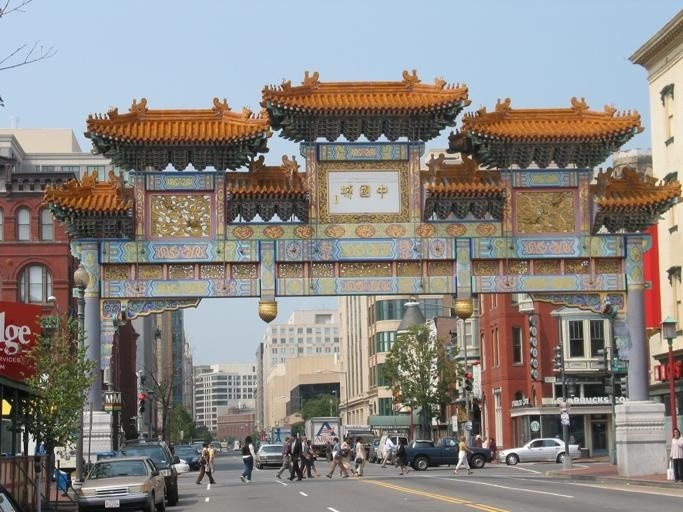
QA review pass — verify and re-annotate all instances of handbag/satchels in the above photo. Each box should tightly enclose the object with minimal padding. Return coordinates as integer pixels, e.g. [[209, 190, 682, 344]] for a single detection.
[[356, 458, 363, 463], [302, 452, 313, 463], [199, 457, 206, 465], [333, 449, 348, 459]]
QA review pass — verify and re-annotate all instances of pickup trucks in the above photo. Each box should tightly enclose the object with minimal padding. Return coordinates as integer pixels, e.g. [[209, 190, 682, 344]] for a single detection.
[[400, 436, 495, 472]]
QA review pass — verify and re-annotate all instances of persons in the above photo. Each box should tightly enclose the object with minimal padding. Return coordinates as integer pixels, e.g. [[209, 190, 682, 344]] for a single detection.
[[488, 438, 496, 461], [275, 430, 409, 481], [473, 438, 482, 448], [240, 436, 258, 483], [195, 443, 216, 484], [669, 427, 683, 482], [453, 436, 474, 475]]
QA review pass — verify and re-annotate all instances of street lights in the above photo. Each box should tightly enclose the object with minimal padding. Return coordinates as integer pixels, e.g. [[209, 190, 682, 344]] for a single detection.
[[658, 316, 680, 436], [72, 264, 90, 483]]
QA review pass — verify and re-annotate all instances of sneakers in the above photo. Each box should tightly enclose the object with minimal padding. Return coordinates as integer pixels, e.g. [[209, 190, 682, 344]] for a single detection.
[[276, 474, 321, 481], [241, 476, 254, 482], [326, 469, 363, 478], [210, 482, 217, 484]]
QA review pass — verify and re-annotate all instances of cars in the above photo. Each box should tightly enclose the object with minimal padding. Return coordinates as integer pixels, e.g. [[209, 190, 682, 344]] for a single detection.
[[74, 455, 169, 512], [255, 443, 283, 471], [497, 436, 584, 466], [172, 441, 208, 470], [347, 430, 408, 464]]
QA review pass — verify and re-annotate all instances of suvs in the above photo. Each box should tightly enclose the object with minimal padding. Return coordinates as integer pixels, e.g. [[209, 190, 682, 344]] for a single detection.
[[109, 438, 180, 505]]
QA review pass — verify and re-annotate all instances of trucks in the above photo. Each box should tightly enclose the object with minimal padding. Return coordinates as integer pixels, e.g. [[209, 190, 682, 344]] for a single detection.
[[305, 415, 342, 460]]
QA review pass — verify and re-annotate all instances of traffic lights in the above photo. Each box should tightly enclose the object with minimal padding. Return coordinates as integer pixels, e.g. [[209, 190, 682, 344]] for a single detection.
[[597, 349, 609, 375], [136, 391, 147, 413], [464, 371, 473, 394]]
